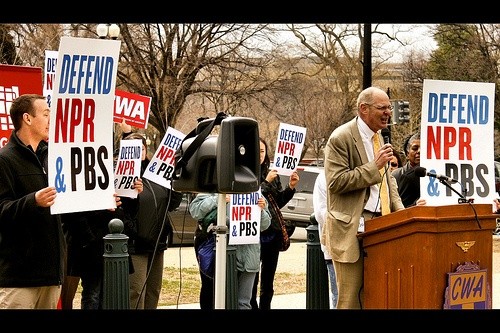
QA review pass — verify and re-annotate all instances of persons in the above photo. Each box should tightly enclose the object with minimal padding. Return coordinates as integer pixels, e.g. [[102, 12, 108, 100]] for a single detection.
[[60, 118, 184, 309], [313, 133, 500, 309], [250, 137, 300, 309], [0, 94, 122, 309], [189, 192, 272, 309], [324, 87, 406, 309]]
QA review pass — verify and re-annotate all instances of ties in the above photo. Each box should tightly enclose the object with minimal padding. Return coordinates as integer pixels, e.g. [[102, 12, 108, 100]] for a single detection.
[[372, 133, 391, 216]]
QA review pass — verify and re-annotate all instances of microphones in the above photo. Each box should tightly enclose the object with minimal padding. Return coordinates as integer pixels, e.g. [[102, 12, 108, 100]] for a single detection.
[[381, 128, 391, 166], [415, 167, 458, 183]]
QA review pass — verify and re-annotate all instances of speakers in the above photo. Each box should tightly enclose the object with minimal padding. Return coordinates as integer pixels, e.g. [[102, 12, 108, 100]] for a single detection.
[[170, 132, 219, 193], [212, 116, 260, 195]]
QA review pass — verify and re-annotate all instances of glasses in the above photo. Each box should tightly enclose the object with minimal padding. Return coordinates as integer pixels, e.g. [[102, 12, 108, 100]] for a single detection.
[[364, 102, 394, 111]]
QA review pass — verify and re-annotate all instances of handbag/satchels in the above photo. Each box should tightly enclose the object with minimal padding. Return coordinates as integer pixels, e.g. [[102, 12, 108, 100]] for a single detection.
[[279, 225, 290, 252]]
